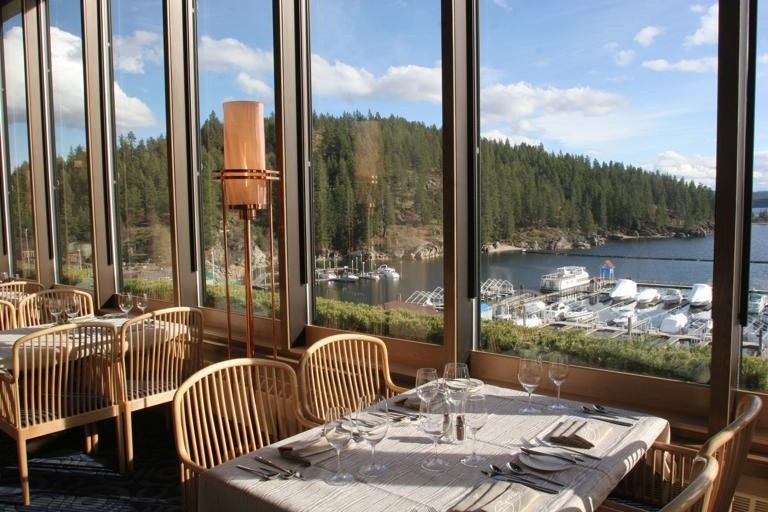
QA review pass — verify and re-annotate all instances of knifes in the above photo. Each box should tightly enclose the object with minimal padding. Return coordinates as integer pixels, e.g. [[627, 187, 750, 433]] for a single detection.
[[480, 470, 559, 494], [254, 454, 306, 482]]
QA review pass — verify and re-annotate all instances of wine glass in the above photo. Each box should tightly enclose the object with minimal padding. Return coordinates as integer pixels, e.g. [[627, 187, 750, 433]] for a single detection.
[[323, 406, 354, 488], [414, 362, 490, 474], [548, 351, 570, 410], [48, 293, 81, 325], [354, 393, 390, 478], [118, 290, 149, 320], [516, 348, 545, 415]]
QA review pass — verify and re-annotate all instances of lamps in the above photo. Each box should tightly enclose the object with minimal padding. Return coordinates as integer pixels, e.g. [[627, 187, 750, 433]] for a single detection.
[[213, 98, 283, 358]]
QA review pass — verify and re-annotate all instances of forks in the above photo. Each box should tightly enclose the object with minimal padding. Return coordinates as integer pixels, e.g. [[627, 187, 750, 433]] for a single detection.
[[519, 435, 603, 462]]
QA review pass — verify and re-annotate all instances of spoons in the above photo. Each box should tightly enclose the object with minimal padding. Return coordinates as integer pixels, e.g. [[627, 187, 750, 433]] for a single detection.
[[489, 463, 536, 485], [580, 402, 639, 428], [237, 463, 296, 481], [506, 460, 564, 488], [366, 405, 427, 426]]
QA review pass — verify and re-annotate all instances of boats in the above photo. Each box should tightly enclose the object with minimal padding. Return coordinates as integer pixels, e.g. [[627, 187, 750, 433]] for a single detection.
[[513, 266, 768, 334], [318, 264, 400, 281]]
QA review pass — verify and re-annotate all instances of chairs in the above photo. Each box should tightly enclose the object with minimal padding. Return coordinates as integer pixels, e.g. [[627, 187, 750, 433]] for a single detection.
[[173, 334, 763, 511], [0, 281, 203, 507]]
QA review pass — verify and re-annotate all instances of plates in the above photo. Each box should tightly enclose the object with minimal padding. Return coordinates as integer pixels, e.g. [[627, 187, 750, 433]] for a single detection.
[[418, 376, 485, 404], [518, 446, 576, 473]]
